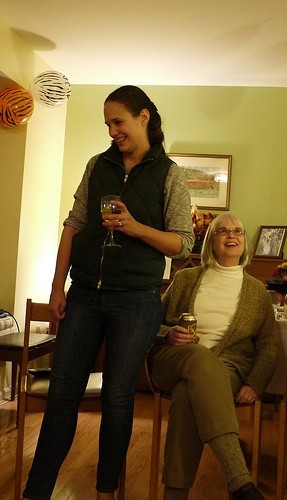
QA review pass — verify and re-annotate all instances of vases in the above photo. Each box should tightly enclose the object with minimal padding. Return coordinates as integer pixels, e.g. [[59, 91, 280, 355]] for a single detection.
[[195, 240, 203, 252]]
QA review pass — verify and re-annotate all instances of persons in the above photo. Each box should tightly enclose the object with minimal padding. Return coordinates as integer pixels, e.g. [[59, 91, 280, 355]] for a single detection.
[[273, 262, 286, 307], [18, 84, 196, 500], [263, 231, 279, 257], [144, 211, 278, 500]]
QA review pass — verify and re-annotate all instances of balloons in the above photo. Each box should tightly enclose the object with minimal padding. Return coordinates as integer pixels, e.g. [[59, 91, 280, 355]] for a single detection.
[[0, 84, 34, 129], [28, 70, 72, 109]]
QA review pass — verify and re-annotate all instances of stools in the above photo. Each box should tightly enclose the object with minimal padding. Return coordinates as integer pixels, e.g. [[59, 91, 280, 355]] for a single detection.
[[0, 331, 56, 429]]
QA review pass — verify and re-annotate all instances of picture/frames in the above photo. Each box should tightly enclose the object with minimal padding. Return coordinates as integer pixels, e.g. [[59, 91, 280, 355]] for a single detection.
[[166, 152, 233, 211], [254, 225, 287, 259]]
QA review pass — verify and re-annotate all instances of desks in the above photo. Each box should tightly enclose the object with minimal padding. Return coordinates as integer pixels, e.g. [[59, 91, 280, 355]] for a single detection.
[[264, 306, 287, 395]]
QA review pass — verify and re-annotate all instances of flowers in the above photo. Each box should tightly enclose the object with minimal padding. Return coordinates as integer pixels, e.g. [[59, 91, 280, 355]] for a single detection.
[[190, 205, 218, 240], [265, 262, 287, 297]]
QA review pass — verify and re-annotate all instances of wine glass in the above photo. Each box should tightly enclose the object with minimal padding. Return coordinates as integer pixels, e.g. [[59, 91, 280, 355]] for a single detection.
[[101, 195, 124, 250]]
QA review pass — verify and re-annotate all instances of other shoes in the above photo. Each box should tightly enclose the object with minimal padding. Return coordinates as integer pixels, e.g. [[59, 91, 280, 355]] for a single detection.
[[230, 483, 266, 499]]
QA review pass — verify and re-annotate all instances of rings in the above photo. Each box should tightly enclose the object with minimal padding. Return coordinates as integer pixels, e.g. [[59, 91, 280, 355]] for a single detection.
[[117, 220, 123, 227]]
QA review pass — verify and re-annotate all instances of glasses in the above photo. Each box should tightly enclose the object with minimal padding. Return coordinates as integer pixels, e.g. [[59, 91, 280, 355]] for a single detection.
[[212, 227, 243, 235]]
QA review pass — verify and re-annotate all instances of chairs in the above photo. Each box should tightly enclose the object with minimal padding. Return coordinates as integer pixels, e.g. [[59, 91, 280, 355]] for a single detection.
[[144, 343, 264, 500], [13, 299, 103, 500]]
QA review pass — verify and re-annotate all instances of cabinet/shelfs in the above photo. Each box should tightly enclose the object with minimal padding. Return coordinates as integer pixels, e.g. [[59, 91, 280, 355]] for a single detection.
[[169, 254, 287, 286]]
